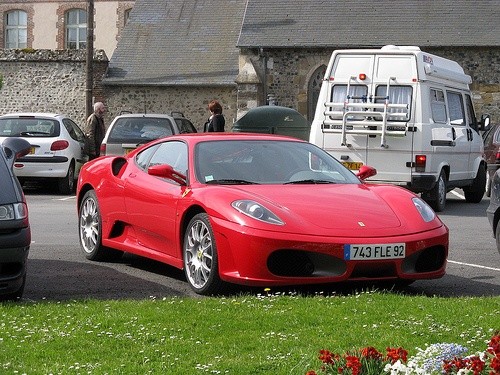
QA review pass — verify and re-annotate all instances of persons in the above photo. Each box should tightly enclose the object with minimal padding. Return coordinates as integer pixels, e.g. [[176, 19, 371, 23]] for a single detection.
[[84, 101, 106, 163], [202, 100, 225, 132]]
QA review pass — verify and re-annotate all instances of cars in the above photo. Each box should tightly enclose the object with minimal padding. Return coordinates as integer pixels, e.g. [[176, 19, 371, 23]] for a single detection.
[[0, 112, 90, 301]]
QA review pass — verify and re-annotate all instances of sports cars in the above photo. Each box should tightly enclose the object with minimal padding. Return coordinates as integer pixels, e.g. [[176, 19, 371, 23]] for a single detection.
[[75, 132, 450, 296]]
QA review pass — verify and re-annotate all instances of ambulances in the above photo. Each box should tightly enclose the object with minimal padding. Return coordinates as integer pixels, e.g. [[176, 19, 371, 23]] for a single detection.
[[308, 44, 500, 255]]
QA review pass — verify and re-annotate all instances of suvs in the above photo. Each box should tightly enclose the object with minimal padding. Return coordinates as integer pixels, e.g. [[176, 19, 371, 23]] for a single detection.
[[100, 110, 198, 156]]
